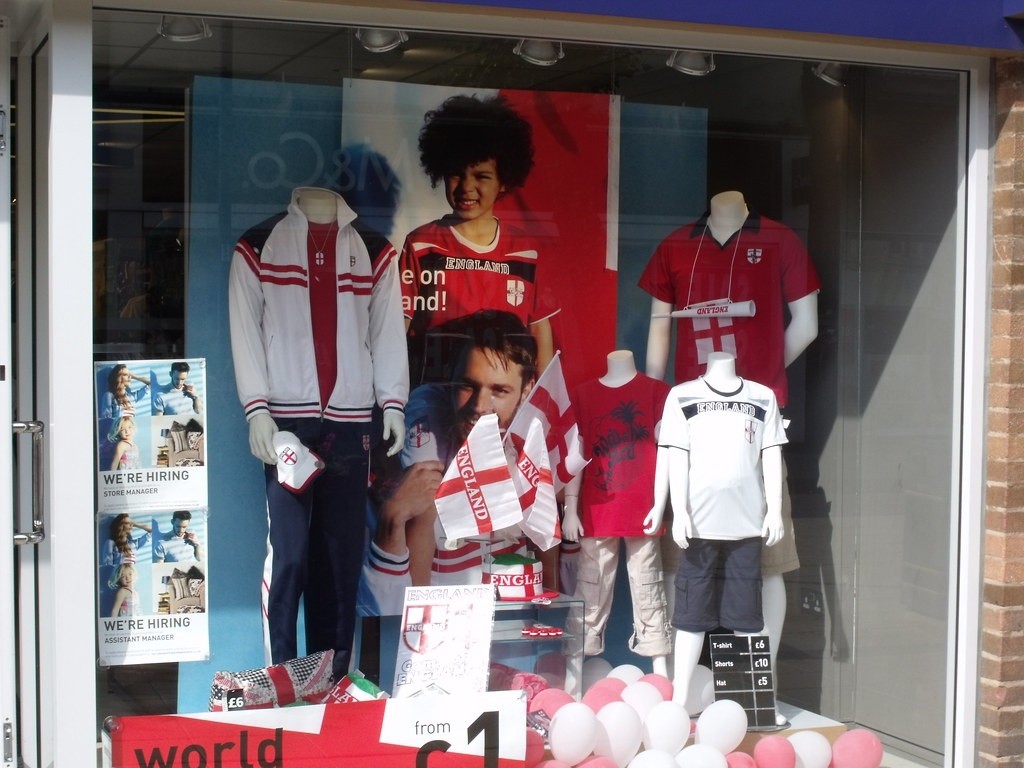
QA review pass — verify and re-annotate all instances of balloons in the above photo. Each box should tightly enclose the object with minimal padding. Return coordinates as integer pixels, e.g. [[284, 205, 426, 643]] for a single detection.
[[488, 649, 882, 768]]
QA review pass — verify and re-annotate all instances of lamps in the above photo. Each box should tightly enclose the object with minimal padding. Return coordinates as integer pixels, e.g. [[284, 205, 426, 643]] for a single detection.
[[157, 15, 213, 41], [665, 49, 715, 76], [513, 39, 565, 66], [354, 29, 408, 52], [810, 61, 848, 88]]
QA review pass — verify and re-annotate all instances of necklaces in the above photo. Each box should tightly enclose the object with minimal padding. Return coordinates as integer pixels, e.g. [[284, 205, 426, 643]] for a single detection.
[[308, 216, 337, 266]]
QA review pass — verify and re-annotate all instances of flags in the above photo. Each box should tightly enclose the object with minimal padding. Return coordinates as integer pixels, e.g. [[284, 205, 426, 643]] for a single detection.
[[511, 417, 562, 552], [503, 348, 593, 495], [434, 413, 525, 539]]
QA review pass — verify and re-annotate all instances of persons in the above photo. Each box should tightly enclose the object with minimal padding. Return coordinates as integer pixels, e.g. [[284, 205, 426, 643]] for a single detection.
[[153, 362, 203, 416], [639, 190, 814, 696], [107, 563, 144, 617], [154, 510, 203, 563], [102, 513, 152, 565], [227, 186, 411, 683], [641, 353, 788, 725], [100, 364, 151, 419], [560, 348, 673, 703], [107, 416, 141, 471], [355, 95, 583, 617]]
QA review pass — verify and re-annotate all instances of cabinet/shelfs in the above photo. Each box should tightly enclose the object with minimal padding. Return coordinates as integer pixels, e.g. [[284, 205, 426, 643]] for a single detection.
[[490, 592, 586, 703]]
